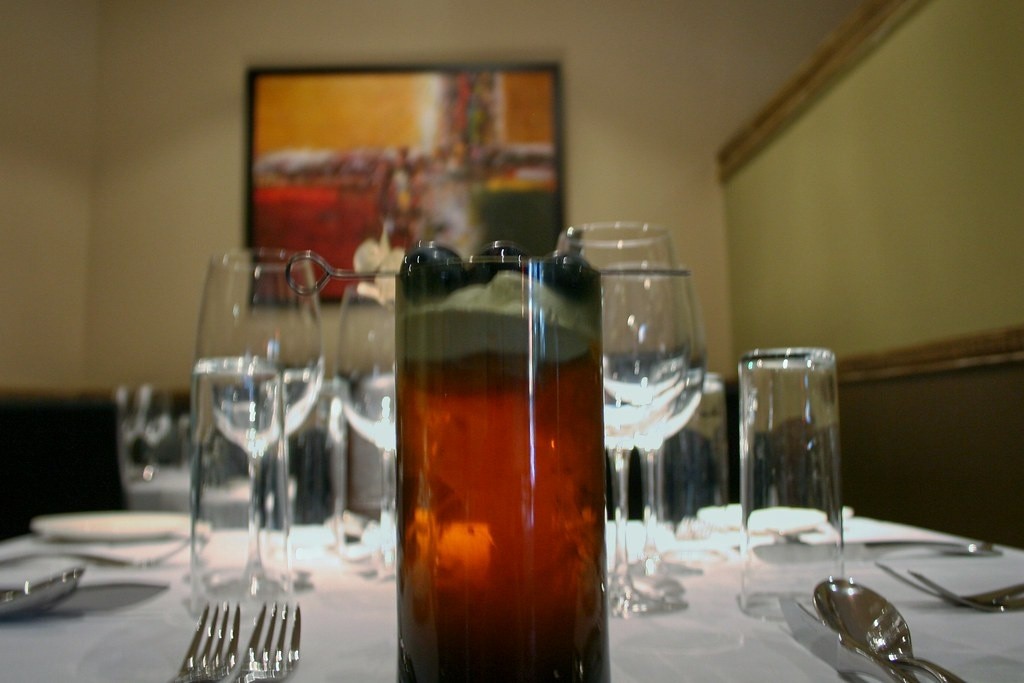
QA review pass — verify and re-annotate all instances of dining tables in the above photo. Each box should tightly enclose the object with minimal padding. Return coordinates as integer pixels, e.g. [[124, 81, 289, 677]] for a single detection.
[[2, 508, 1023, 682]]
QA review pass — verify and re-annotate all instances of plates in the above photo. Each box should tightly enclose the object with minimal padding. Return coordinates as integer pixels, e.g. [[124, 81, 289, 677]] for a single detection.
[[29, 508, 207, 547]]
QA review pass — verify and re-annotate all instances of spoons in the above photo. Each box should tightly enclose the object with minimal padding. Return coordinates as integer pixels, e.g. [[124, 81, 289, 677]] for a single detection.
[[815, 580, 964, 682]]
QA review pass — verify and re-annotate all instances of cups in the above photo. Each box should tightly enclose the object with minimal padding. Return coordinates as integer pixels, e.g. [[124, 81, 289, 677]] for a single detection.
[[188, 360, 293, 602], [647, 370, 731, 561], [115, 381, 193, 554], [393, 259, 614, 683], [739, 347, 844, 622]]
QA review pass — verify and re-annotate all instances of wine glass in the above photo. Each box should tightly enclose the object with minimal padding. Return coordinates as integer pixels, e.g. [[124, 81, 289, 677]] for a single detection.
[[336, 278, 397, 582], [593, 263, 705, 586], [196, 250, 328, 600], [551, 223, 691, 617]]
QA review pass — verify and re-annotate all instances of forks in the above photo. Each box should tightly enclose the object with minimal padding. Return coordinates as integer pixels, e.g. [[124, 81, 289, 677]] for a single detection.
[[233, 603, 304, 683], [168, 603, 241, 682], [794, 597, 920, 683], [874, 560, 1023, 613]]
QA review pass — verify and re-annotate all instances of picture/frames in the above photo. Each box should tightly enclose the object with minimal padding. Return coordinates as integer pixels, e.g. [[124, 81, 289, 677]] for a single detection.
[[245, 61, 567, 310]]
